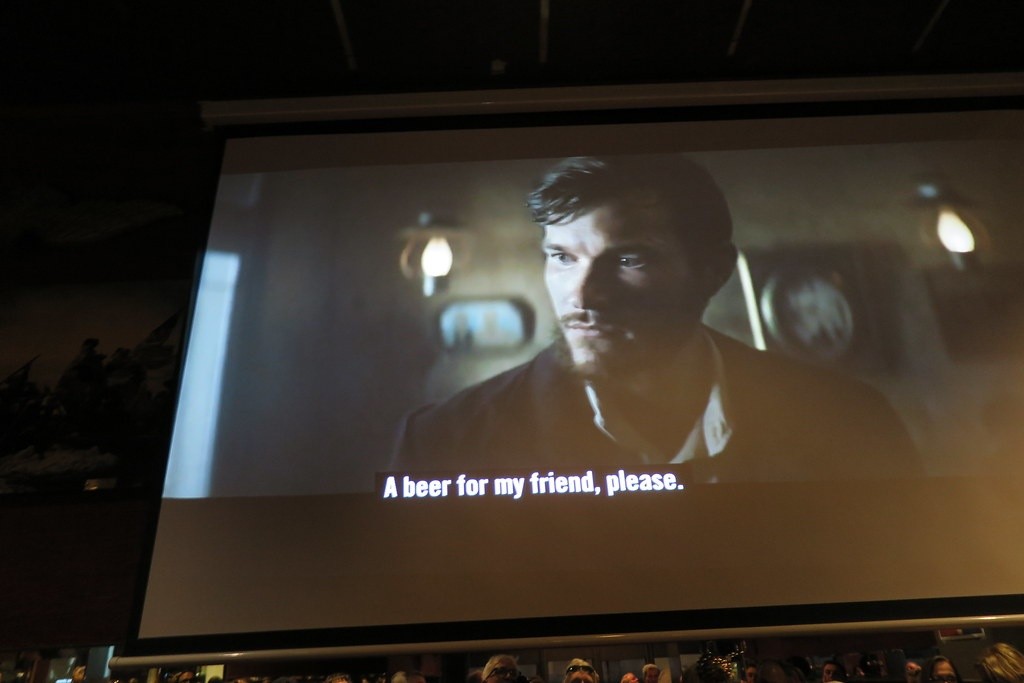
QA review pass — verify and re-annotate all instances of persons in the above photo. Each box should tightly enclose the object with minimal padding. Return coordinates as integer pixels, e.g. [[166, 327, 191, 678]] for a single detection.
[[162, 643, 1023, 683], [388, 153, 925, 489]]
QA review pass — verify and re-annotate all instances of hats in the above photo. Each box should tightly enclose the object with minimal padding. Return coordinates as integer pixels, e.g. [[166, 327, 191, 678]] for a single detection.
[[786, 655, 810, 676]]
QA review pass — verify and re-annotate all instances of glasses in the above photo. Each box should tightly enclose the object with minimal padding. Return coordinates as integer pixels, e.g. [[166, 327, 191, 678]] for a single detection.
[[929, 675, 957, 683], [486, 667, 520, 680], [565, 665, 595, 675]]
